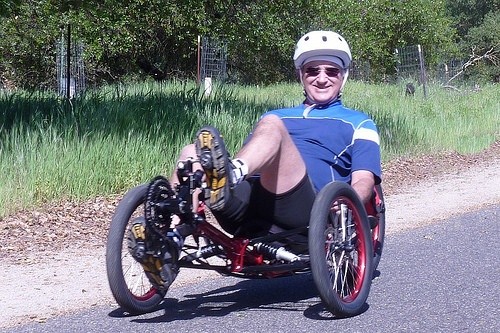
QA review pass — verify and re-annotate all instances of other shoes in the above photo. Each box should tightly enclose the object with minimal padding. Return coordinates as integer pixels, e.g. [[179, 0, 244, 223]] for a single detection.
[[127, 215, 180, 291], [195, 126, 232, 212]]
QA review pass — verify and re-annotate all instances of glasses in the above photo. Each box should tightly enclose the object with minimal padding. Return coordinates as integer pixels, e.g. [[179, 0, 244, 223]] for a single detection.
[[304, 66, 342, 77]]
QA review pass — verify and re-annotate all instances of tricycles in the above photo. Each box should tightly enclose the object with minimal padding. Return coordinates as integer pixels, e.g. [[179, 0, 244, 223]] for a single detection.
[[105, 159, 385, 318]]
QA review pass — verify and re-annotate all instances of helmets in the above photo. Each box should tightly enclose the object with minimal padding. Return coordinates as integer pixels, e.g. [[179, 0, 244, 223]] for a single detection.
[[293, 31, 352, 70]]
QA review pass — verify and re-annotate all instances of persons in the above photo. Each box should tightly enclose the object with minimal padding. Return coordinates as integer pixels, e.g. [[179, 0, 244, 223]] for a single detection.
[[127, 31, 382, 291]]
